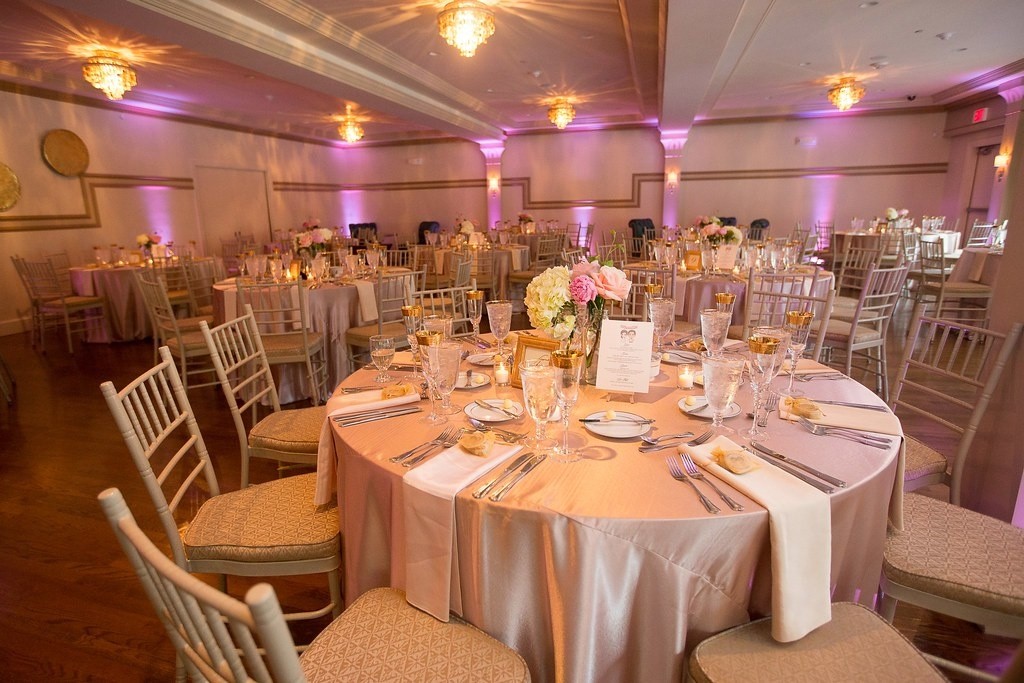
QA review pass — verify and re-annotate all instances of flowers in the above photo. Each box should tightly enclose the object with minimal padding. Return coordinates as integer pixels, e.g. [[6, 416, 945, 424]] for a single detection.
[[884, 206, 908, 223], [693, 214, 738, 246], [518, 213, 535, 226], [292, 218, 333, 255], [458, 219, 481, 236], [523, 231, 633, 378]]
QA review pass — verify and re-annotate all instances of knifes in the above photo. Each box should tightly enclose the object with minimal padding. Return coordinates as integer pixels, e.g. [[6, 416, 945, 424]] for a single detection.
[[659, 350, 699, 361], [474, 399, 519, 418], [332, 406, 424, 427], [741, 440, 846, 494], [464, 369, 472, 389], [686, 403, 709, 415], [472, 452, 547, 502]]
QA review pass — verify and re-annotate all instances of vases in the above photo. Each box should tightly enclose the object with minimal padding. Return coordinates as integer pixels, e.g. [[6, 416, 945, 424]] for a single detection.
[[303, 245, 326, 277], [557, 305, 602, 384], [706, 244, 724, 273]]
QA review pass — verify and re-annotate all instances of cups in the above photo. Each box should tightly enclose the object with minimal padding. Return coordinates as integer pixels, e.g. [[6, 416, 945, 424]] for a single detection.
[[677, 364, 696, 390]]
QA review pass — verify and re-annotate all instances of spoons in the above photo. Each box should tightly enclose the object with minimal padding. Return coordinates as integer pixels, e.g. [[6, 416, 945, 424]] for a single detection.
[[746, 410, 755, 419], [640, 432, 694, 444]]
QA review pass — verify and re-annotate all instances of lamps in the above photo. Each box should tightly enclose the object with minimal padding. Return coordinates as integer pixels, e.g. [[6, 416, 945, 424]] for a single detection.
[[437, 0, 495, 58], [337, 117, 365, 144], [993, 153, 1008, 181], [827, 77, 864, 112], [667, 170, 678, 196], [82, 49, 137, 102], [489, 177, 499, 199], [547, 97, 576, 129]]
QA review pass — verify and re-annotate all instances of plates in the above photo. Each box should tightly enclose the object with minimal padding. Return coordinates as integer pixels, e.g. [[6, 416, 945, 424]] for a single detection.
[[578, 418, 655, 425], [678, 395, 742, 422], [657, 349, 701, 365], [451, 351, 524, 423], [692, 370, 745, 387], [584, 411, 652, 439]]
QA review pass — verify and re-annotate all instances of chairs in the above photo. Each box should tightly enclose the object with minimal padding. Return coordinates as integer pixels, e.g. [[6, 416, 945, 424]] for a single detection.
[[98, 488, 532, 683], [10, 218, 1008, 408], [888, 304, 1022, 507], [99, 346, 345, 682], [686, 602, 950, 682], [199, 305, 325, 488], [881, 490, 1024, 683]]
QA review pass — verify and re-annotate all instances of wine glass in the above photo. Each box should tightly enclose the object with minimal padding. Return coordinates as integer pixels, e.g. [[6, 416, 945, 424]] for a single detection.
[[850, 214, 947, 238], [645, 284, 676, 353], [700, 350, 747, 438], [737, 308, 814, 442], [401, 304, 463, 427], [519, 349, 585, 464], [700, 291, 737, 362], [465, 291, 512, 368], [424, 218, 559, 254], [647, 233, 802, 277], [369, 334, 396, 383], [234, 244, 386, 288]]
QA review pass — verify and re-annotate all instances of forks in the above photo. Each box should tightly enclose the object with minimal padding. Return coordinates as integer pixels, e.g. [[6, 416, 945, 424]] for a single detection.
[[390, 426, 466, 467], [665, 452, 745, 514], [784, 369, 850, 382], [340, 380, 403, 394], [757, 394, 776, 427], [638, 430, 714, 454], [797, 414, 892, 450]]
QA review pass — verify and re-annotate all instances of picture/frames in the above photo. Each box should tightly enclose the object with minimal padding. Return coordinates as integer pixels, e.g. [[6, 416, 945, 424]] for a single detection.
[[510, 335, 560, 388]]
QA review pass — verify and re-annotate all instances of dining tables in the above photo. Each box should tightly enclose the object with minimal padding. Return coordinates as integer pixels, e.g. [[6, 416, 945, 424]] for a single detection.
[[213, 266, 417, 406], [683, 240, 798, 268], [832, 231, 961, 289], [412, 242, 529, 290], [69, 257, 222, 342], [623, 257, 836, 328], [486, 231, 572, 264], [937, 250, 1001, 332]]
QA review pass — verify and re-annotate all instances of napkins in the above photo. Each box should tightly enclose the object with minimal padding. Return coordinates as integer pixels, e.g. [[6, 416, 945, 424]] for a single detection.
[[223, 249, 906, 642]]
